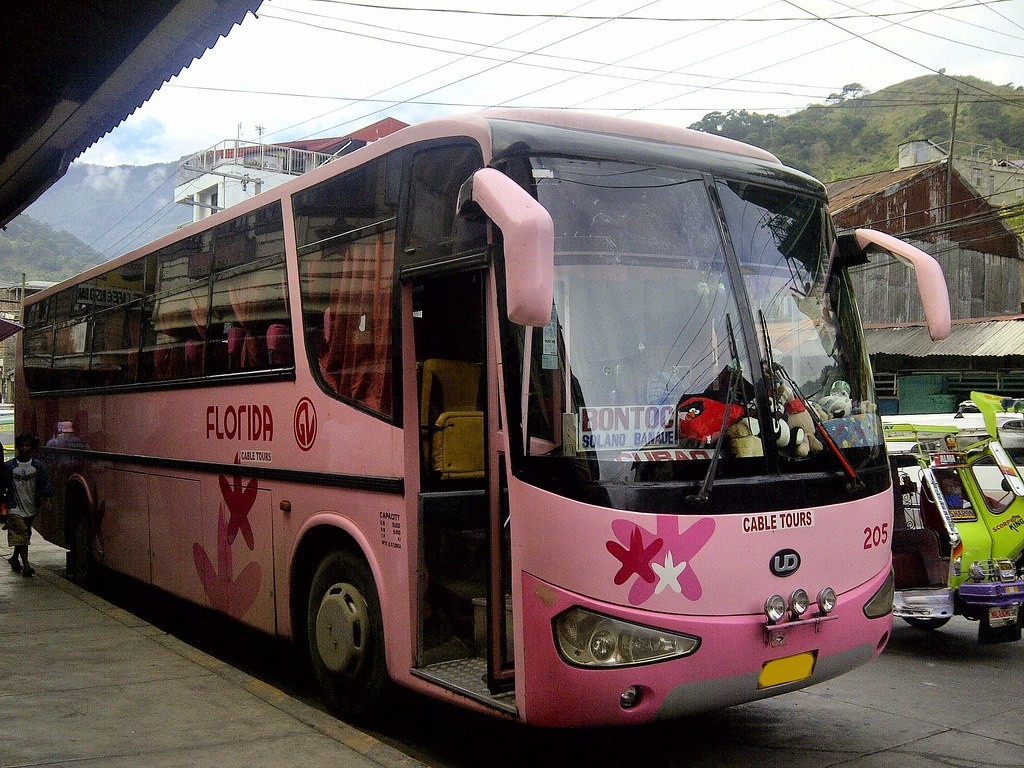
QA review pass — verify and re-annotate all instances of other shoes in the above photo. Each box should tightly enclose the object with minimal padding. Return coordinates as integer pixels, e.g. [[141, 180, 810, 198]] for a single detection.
[[8, 556, 22, 572], [23, 565, 35, 577]]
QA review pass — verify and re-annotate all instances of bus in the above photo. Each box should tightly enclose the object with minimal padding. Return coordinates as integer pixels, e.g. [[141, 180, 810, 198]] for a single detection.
[[14, 107, 950, 727]]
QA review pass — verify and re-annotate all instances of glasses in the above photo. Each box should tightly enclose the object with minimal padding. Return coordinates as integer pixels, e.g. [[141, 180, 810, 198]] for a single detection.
[[942, 475, 952, 479]]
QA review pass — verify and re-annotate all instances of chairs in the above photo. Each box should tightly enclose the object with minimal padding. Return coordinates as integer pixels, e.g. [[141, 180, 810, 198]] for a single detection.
[[421, 358, 488, 485], [91, 305, 331, 383]]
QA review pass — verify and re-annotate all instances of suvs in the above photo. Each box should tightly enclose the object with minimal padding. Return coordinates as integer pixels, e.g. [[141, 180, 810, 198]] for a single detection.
[[879, 400, 1024, 530]]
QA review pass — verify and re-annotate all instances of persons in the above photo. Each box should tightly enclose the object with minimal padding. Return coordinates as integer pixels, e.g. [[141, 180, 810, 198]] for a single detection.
[[48, 421, 87, 473], [4, 433, 52, 575]]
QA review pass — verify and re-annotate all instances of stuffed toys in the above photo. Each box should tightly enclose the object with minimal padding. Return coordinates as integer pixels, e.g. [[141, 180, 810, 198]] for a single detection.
[[731, 379, 877, 458]]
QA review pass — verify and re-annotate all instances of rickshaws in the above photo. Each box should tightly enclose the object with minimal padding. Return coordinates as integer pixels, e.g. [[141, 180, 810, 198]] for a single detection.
[[874, 392, 1024, 642]]
